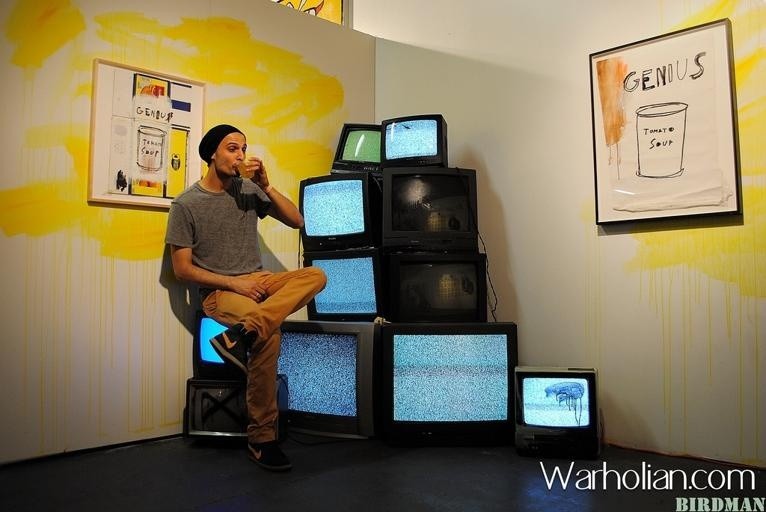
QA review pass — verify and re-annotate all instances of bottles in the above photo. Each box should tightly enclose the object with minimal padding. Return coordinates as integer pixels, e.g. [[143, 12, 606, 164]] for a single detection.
[[199, 124, 246, 165]]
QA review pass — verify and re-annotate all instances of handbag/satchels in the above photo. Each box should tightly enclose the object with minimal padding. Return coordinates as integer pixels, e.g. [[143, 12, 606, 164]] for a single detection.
[[264, 184, 273, 195]]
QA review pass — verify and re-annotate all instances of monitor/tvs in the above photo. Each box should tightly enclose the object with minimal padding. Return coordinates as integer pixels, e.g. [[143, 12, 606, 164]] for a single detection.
[[183, 114, 604, 458]]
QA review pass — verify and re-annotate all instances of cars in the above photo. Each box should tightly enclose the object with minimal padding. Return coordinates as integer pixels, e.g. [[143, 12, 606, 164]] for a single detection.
[[634, 102, 689, 180], [137, 124, 167, 172], [235, 160, 255, 179]]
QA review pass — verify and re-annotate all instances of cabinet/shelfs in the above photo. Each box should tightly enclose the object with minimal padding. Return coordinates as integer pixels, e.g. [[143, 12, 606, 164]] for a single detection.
[[171, 154, 181, 171]]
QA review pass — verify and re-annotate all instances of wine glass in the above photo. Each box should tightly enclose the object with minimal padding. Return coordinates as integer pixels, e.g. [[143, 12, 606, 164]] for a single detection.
[[255, 292, 259, 297]]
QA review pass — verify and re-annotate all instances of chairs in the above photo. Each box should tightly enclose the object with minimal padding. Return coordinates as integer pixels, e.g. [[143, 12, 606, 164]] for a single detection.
[[209, 323, 259, 377], [246, 439, 294, 472]]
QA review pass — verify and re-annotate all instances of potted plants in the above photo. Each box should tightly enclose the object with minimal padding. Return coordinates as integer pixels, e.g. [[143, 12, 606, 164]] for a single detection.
[[587, 19, 742, 227], [273, 0, 353, 28], [84, 56, 206, 211]]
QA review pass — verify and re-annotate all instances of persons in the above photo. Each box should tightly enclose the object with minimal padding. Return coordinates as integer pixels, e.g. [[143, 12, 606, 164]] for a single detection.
[[163, 123, 329, 471]]
